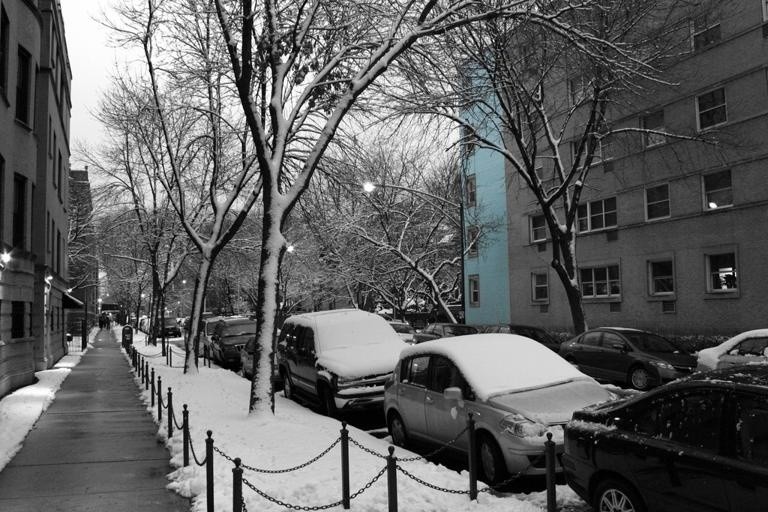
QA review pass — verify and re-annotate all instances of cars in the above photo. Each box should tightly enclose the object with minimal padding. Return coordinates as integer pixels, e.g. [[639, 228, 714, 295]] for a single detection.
[[130, 312, 257, 378]]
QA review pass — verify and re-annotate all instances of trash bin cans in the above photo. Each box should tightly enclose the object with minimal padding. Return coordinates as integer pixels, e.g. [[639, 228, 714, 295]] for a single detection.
[[122, 325, 133, 347]]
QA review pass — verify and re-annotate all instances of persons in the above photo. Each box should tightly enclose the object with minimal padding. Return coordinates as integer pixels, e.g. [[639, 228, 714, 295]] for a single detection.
[[99, 316, 110, 330]]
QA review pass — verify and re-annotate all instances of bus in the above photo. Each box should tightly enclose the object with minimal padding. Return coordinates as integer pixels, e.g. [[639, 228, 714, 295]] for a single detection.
[[101, 303, 122, 315]]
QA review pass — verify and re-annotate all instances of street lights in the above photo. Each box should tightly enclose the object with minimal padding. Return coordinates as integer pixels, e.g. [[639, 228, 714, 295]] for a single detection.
[[364, 182, 466, 325]]
